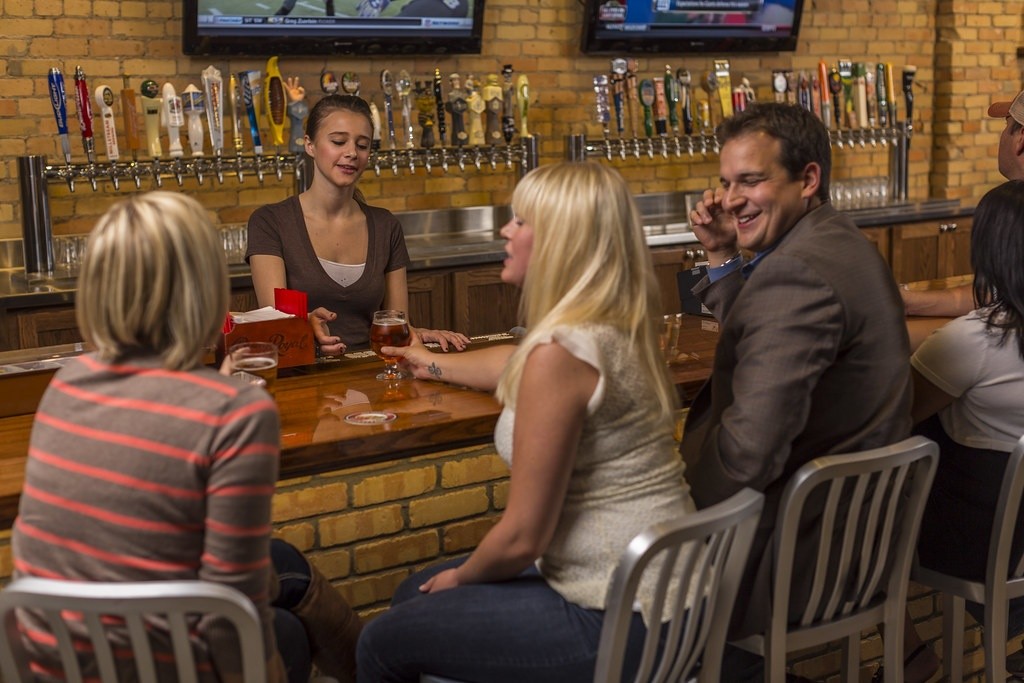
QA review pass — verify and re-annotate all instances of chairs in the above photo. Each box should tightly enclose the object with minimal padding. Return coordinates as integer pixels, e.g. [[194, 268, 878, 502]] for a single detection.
[[722, 435, 939, 683], [589, 487, 765, 683], [0, 575, 266, 683], [899, 431, 1024, 682]]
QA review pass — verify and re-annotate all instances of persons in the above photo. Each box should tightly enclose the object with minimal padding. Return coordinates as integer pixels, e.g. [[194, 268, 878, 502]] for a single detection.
[[678, 103, 913, 683], [12, 192, 364, 683], [871, 180, 1024, 683], [353, 161, 711, 683], [243, 95, 472, 355], [896, 89, 1024, 317], [272, 0, 468, 19]]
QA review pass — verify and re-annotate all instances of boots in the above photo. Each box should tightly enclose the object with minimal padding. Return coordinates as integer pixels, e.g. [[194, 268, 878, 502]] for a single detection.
[[288, 543, 363, 683]]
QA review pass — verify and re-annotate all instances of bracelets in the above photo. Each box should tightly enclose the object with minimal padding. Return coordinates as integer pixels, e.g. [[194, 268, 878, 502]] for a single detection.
[[706, 250, 741, 269]]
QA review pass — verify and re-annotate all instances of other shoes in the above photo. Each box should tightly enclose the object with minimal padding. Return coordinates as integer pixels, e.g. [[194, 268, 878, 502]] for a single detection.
[[1005, 647, 1024, 676], [870, 644, 940, 683]]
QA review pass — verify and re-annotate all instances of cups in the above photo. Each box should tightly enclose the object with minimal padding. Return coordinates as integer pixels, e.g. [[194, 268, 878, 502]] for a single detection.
[[227, 342, 278, 406], [823, 175, 895, 212], [658, 313, 682, 358], [215, 221, 254, 265], [52, 234, 91, 280]]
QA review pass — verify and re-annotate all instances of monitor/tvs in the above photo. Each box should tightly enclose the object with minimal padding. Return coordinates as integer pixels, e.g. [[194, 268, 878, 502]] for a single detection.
[[580, 0, 804, 56], [181, 0, 485, 58]]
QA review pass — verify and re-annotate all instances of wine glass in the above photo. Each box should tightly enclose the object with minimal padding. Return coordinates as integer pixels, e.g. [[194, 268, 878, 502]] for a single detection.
[[370, 310, 409, 380]]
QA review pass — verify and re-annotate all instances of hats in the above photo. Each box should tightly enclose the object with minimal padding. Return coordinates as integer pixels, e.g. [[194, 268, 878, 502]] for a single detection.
[[988, 88, 1024, 126]]
